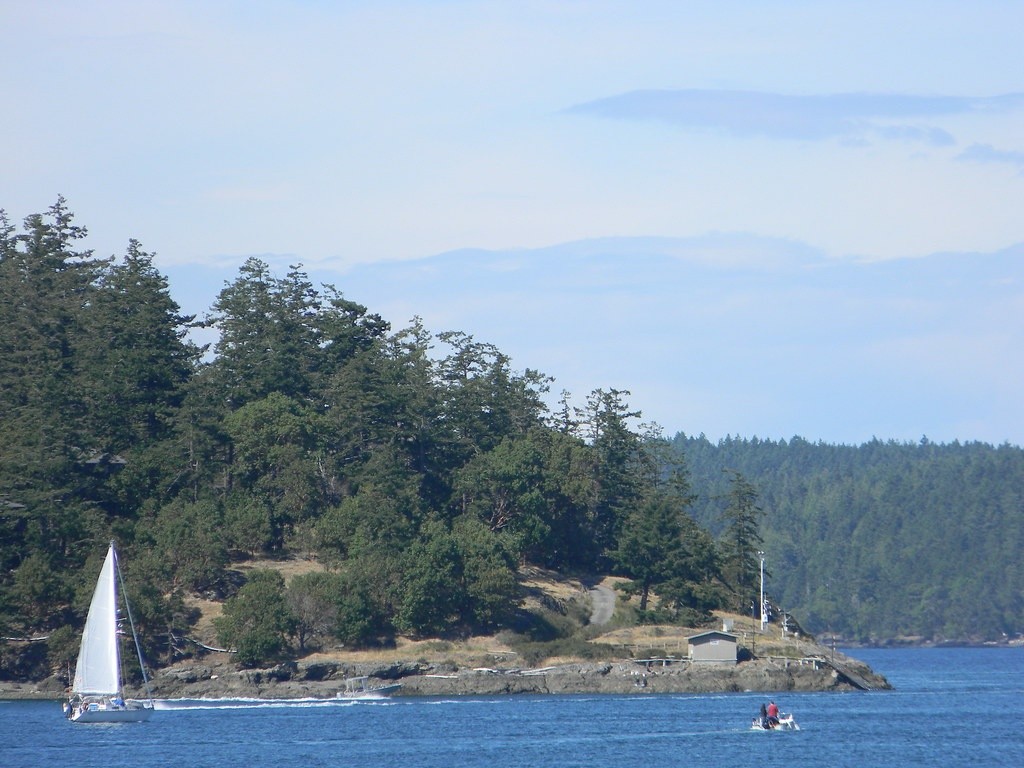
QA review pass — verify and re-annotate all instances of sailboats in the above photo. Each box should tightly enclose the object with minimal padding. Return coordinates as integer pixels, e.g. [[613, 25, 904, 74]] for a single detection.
[[63, 536, 156, 723]]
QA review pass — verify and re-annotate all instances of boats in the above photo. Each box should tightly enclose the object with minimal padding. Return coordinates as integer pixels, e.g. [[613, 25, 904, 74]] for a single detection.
[[750, 710, 801, 733]]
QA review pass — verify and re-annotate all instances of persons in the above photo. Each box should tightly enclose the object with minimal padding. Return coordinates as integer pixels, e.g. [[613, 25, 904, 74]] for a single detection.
[[761, 701, 779, 727], [63, 701, 72, 718]]
